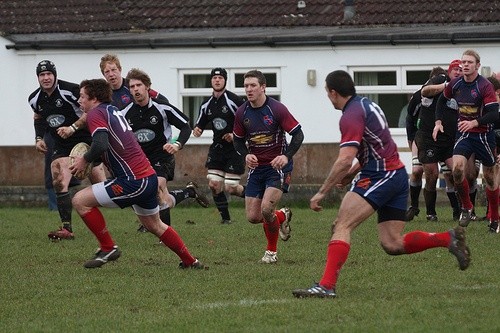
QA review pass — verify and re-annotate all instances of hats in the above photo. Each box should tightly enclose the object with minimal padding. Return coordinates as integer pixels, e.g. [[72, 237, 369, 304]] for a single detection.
[[209, 67, 228, 80], [35, 59, 58, 74], [447, 60, 463, 76]]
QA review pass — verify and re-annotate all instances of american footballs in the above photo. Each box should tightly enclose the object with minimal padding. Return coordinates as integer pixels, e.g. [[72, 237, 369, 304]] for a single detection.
[[68, 142, 95, 180]]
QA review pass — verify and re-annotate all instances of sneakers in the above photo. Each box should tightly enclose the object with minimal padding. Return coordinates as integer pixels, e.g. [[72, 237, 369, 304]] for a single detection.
[[257, 248, 278, 265], [458, 205, 474, 228], [289, 284, 337, 301], [278, 207, 294, 242], [405, 206, 417, 220], [449, 225, 473, 272], [83, 246, 123, 268], [469, 212, 480, 222], [220, 219, 234, 227], [487, 220, 499, 235], [186, 181, 210, 209], [48, 227, 76, 242], [177, 259, 203, 271], [451, 209, 460, 222], [425, 213, 438, 220]]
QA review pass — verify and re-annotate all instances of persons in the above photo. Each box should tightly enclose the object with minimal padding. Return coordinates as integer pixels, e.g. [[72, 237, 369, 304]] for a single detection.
[[419, 59, 465, 221], [69, 78, 206, 273], [293, 70, 473, 299], [405, 67, 463, 221], [98, 52, 169, 234], [115, 66, 209, 243], [192, 67, 248, 225], [484, 75, 499, 222], [28, 59, 93, 241], [233, 69, 305, 264], [431, 49, 500, 233]]
